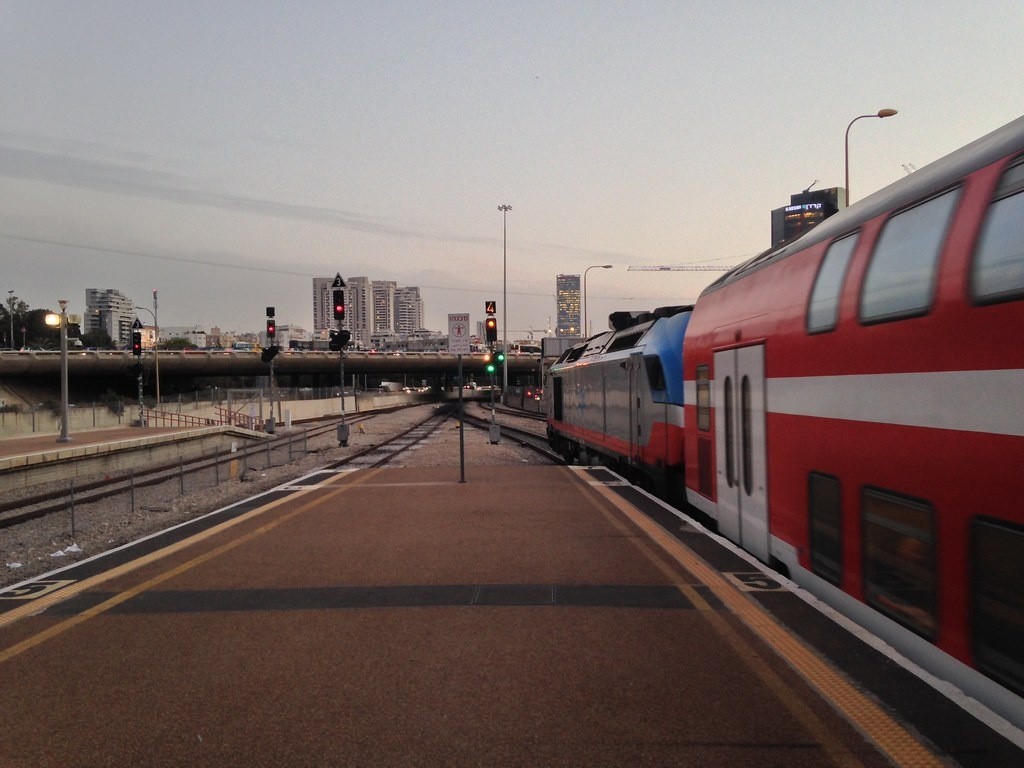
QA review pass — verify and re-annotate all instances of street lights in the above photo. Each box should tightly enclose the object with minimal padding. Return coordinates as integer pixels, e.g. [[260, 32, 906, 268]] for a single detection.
[[496, 205, 513, 392], [8, 290, 14, 350], [583, 264, 614, 341], [134, 289, 160, 407], [44, 298, 82, 444], [844, 108, 898, 209]]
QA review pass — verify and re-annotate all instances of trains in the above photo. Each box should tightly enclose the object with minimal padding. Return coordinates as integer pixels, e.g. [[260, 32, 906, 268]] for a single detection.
[[538, 115, 1023, 756]]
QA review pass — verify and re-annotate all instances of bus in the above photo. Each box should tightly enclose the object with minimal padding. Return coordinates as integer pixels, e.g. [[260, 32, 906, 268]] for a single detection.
[[288, 338, 358, 354], [510, 344, 542, 356], [231, 342, 263, 352]]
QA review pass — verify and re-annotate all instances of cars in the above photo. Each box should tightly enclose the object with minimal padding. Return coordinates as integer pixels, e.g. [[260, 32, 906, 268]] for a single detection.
[[361, 348, 407, 356], [148, 344, 224, 354], [85, 347, 100, 350]]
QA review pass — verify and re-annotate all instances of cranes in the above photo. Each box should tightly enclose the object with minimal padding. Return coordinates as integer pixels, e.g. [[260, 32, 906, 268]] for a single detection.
[[628, 263, 740, 272]]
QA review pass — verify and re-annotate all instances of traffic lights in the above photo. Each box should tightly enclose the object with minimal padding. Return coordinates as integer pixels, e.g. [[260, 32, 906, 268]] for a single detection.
[[267, 320, 276, 338], [492, 351, 505, 367], [133, 332, 141, 355], [486, 318, 497, 341], [485, 362, 496, 374], [262, 346, 279, 362], [333, 290, 345, 321], [329, 330, 350, 351]]
